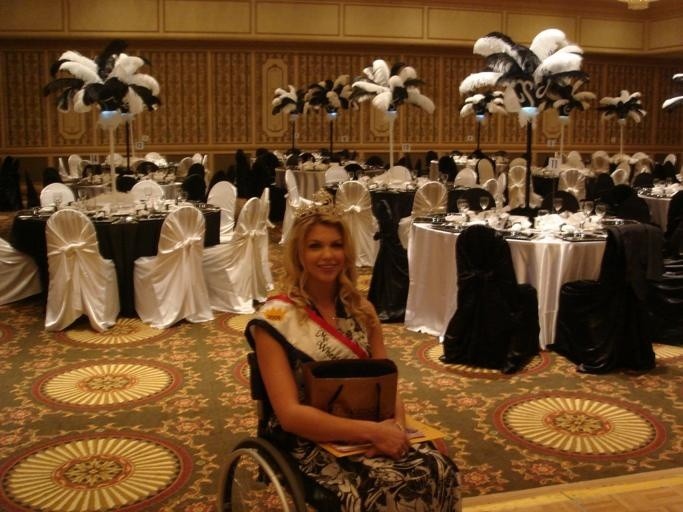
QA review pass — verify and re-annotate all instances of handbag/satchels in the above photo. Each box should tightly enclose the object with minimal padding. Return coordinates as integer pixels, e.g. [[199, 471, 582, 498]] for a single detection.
[[304, 359, 397, 445]]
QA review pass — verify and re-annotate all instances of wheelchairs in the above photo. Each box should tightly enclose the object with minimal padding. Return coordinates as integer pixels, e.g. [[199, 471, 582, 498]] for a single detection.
[[217, 297, 449, 511]]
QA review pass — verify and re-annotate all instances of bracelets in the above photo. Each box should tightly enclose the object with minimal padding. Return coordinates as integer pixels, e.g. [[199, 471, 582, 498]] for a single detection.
[[395, 422, 404, 431]]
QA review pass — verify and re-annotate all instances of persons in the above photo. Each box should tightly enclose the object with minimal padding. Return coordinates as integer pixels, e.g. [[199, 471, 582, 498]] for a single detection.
[[243, 212, 464, 512]]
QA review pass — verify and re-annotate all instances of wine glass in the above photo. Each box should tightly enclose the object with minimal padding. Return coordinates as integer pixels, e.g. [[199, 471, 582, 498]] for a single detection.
[[53, 185, 188, 214], [550, 197, 605, 230], [455, 194, 488, 214], [652, 177, 671, 194], [536, 210, 550, 236], [345, 168, 448, 188]]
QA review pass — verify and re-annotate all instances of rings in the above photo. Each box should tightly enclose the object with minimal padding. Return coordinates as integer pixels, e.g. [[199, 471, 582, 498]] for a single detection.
[[400, 451, 405, 457]]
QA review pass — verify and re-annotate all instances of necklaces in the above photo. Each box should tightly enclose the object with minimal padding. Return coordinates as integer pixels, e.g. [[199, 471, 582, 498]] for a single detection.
[[322, 310, 338, 320]]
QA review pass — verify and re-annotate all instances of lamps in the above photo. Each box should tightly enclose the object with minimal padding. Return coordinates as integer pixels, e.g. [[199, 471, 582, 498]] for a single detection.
[[614, 0, 661, 11]]
